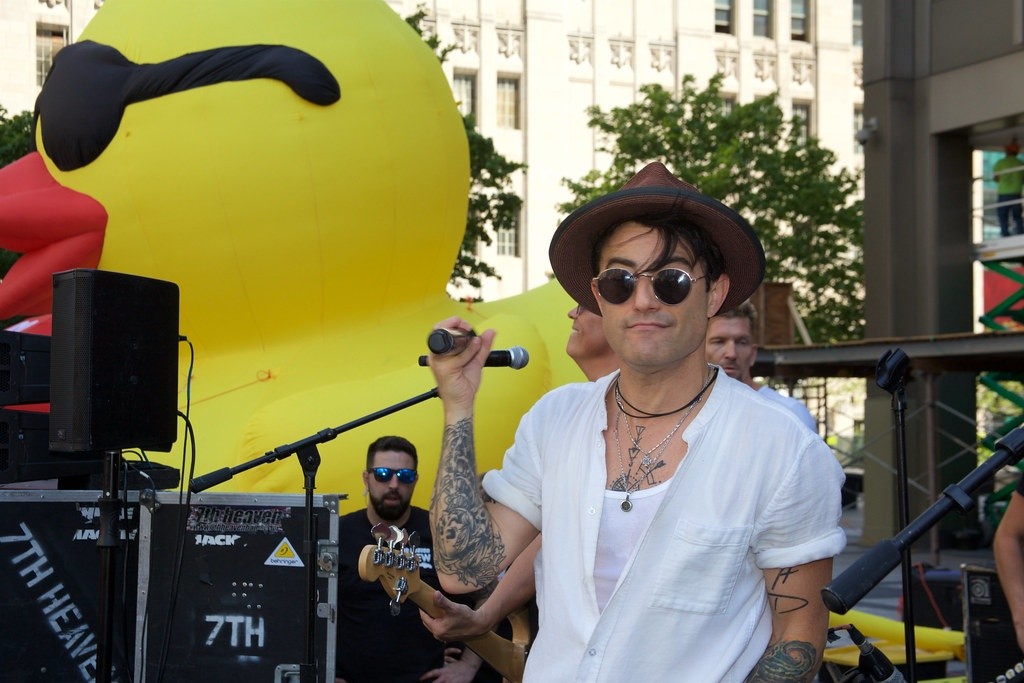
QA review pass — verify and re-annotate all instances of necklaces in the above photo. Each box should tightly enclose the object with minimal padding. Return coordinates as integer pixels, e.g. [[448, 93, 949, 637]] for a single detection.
[[614, 406, 696, 512], [615, 368, 719, 419], [615, 388, 696, 468]]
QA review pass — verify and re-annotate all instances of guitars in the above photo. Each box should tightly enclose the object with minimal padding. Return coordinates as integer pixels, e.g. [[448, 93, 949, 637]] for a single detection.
[[358, 522, 538, 683]]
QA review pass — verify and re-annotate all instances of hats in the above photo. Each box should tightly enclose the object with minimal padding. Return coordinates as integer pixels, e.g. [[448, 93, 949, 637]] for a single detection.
[[547, 162, 766, 316]]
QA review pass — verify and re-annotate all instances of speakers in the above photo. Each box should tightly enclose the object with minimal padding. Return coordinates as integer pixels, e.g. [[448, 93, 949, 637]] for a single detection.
[[961, 560, 1024, 683], [0, 268, 179, 491]]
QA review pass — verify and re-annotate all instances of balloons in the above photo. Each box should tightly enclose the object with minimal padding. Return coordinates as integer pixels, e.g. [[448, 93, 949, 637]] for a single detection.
[[1, 0, 589, 515]]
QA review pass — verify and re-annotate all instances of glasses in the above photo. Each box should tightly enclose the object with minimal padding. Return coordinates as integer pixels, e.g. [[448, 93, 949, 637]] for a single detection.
[[576, 303, 586, 315], [368, 467, 417, 485], [592, 268, 706, 306]]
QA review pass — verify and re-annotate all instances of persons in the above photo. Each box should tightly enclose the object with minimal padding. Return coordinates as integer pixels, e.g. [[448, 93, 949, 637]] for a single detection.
[[427, 161, 848, 683], [336, 435, 495, 682], [419, 304, 628, 640], [707, 299, 817, 434], [993, 477, 1024, 652]]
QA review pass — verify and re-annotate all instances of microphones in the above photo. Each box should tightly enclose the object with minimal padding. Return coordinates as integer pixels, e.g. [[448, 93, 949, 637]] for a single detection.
[[419, 346, 530, 369], [428, 327, 476, 355], [847, 624, 906, 683]]
